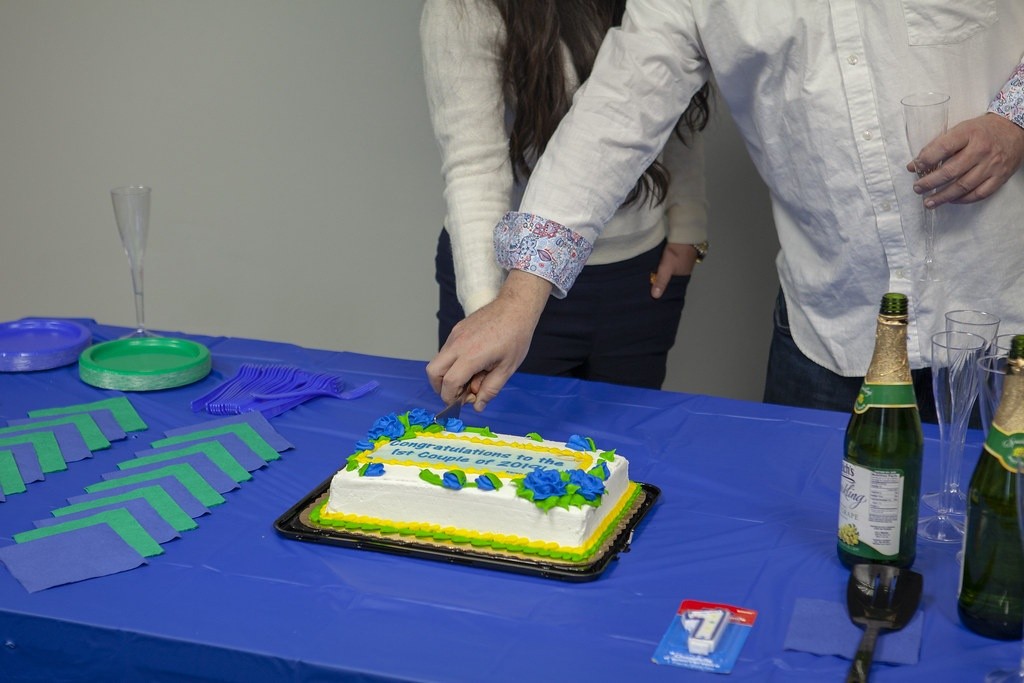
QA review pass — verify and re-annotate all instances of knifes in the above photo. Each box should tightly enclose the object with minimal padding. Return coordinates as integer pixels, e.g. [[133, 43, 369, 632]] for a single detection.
[[432, 380, 473, 428]]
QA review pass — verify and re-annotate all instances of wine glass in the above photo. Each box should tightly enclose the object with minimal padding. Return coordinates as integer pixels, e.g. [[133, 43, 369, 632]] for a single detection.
[[108, 185, 163, 341], [902, 85, 951, 284], [915, 308, 1017, 564]]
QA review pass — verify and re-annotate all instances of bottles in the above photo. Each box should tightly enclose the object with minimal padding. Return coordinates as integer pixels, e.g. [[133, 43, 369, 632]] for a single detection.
[[954, 333, 1024, 644], [834, 293, 927, 574]]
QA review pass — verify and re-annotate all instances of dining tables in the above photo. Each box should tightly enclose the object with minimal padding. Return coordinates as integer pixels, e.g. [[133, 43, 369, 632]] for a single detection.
[[0, 310, 1024, 682]]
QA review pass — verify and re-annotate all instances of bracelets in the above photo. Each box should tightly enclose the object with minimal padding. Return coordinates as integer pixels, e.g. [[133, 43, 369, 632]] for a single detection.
[[693, 238, 710, 265]]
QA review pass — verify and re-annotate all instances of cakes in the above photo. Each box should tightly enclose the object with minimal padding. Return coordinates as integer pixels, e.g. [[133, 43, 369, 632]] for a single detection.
[[309, 409, 642, 562]]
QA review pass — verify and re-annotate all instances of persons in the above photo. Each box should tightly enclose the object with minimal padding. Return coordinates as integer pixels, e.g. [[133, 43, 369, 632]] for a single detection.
[[425, 0, 1024, 425], [412, 0, 714, 395]]
